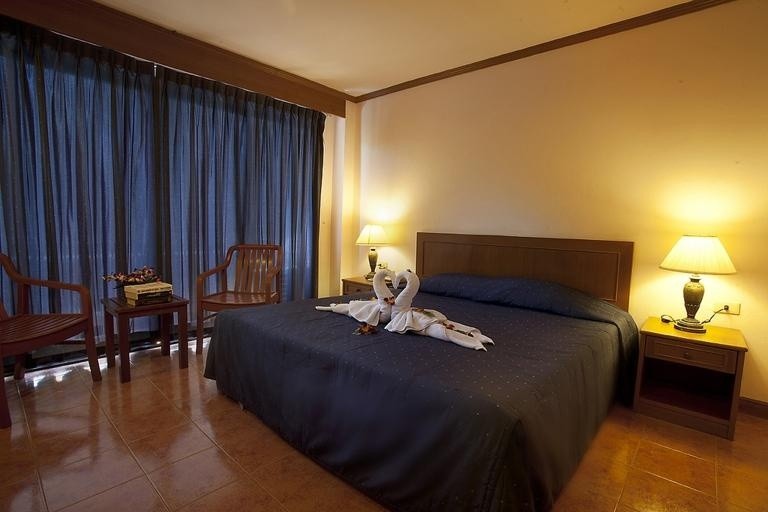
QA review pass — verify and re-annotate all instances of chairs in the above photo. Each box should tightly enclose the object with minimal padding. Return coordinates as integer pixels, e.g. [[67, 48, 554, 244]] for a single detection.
[[194, 244, 285, 354], [0, 251, 105, 426]]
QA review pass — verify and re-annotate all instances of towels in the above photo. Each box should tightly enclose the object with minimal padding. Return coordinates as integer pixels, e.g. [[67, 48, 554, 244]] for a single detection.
[[315, 267, 395, 326], [385, 270, 494, 350]]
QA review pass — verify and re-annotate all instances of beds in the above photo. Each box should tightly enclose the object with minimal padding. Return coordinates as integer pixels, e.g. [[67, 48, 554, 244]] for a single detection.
[[208, 229, 637, 512]]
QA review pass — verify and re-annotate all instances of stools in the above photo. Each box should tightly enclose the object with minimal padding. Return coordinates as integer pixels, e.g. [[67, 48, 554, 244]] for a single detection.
[[99, 297, 190, 383]]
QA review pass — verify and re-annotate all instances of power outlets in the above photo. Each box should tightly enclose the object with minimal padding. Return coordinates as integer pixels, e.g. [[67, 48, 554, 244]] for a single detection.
[[707, 296, 737, 321]]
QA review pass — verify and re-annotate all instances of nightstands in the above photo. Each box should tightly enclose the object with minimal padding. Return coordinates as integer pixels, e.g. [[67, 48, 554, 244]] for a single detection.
[[632, 316, 751, 445], [340, 275, 397, 294]]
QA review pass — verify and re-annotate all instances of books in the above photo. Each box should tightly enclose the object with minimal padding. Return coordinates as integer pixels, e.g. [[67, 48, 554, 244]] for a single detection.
[[123, 282, 173, 307]]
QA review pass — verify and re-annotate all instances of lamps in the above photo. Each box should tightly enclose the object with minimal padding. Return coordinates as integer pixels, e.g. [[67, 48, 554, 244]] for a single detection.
[[657, 235, 738, 333], [355, 221, 399, 282]]
